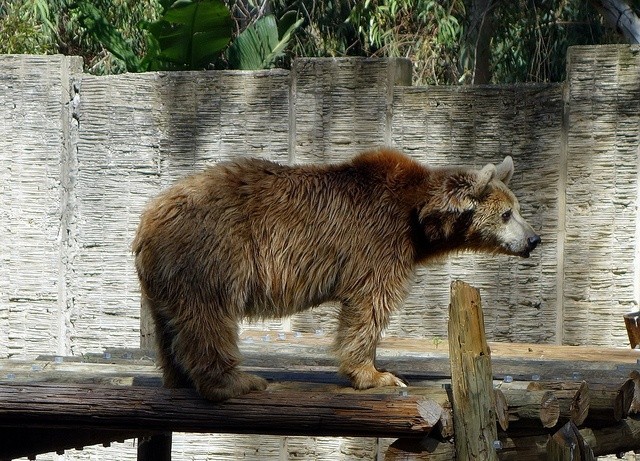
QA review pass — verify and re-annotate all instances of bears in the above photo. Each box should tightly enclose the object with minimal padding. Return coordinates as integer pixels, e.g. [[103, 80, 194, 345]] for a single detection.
[[130, 146, 541, 399]]
